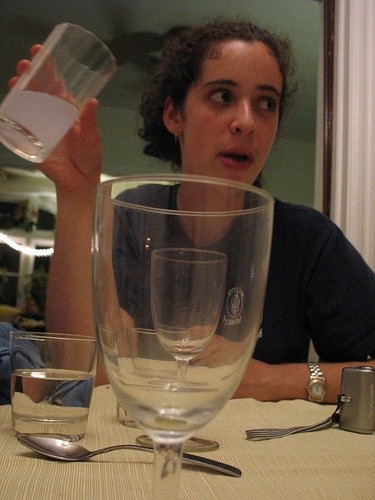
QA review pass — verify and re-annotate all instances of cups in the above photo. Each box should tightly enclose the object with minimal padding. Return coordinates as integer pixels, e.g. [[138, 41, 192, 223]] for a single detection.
[[125, 326, 186, 378], [117, 402, 143, 428], [9, 331, 99, 442], [0, 23, 119, 165]]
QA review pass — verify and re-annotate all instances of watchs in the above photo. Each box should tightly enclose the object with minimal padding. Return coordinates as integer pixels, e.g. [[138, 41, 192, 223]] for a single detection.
[[306, 363, 327, 403]]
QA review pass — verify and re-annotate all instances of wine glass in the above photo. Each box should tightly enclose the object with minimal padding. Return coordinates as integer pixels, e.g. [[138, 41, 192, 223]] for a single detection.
[[93, 172, 275, 500], [144, 247, 226, 393], [148, 247, 229, 387]]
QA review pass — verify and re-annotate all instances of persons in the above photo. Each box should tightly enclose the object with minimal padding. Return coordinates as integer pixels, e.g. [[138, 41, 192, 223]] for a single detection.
[[8, 15, 375, 405]]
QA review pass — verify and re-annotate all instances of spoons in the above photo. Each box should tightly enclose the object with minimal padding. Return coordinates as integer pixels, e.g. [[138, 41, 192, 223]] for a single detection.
[[16, 435, 243, 478]]
